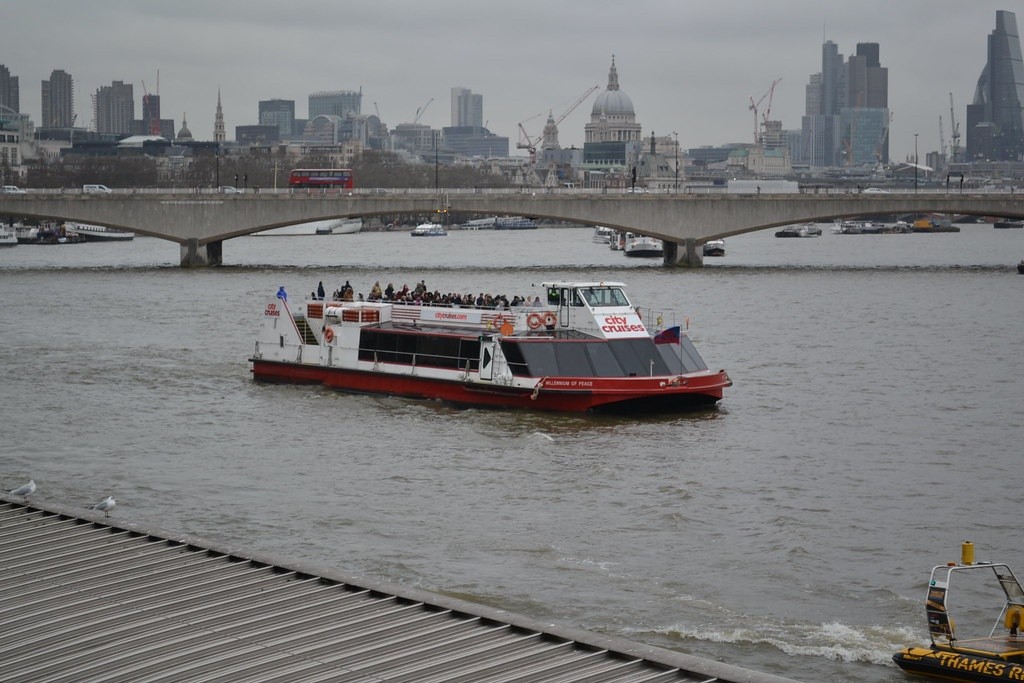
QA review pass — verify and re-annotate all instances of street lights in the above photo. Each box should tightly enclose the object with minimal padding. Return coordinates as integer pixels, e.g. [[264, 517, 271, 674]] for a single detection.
[[914, 132, 920, 195]]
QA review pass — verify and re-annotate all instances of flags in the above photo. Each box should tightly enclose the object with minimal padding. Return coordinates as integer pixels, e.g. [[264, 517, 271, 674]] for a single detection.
[[654, 325, 680, 345]]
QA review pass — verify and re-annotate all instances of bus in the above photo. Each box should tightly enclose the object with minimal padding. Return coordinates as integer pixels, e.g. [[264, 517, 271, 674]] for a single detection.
[[287, 169, 354, 197]]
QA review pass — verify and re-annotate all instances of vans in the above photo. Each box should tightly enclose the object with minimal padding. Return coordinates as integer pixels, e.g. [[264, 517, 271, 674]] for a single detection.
[[83, 185, 113, 194], [2, 186, 26, 194]]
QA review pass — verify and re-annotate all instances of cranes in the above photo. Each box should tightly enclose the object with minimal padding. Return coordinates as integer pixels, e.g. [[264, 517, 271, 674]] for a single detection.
[[416, 97, 436, 124], [515, 85, 602, 154], [939, 115, 949, 156], [879, 112, 897, 147], [141, 80, 149, 102], [948, 92, 962, 163], [748, 76, 784, 141]]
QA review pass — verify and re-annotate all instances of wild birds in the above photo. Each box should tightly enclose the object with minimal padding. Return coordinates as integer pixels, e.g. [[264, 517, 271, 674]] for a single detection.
[[85, 495, 116, 518], [8, 479, 36, 503]]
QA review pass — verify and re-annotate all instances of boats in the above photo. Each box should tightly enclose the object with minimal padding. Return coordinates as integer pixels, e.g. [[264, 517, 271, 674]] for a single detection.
[[315, 229, 334, 234], [702, 238, 726, 257], [0, 219, 136, 245], [829, 218, 961, 233], [411, 222, 448, 237], [246, 277, 735, 414], [994, 221, 1024, 228], [495, 215, 538, 229], [1018, 260, 1024, 274], [462, 217, 496, 231], [775, 223, 823, 240], [592, 225, 664, 254]]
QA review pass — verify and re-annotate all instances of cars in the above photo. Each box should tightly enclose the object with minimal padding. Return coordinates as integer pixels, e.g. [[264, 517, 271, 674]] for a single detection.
[[863, 188, 887, 195], [218, 186, 240, 194]]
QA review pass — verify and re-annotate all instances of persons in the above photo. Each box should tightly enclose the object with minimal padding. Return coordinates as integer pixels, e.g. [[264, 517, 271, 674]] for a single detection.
[[369, 281, 382, 300], [277, 286, 287, 301], [318, 281, 325, 300], [549, 290, 559, 302], [311, 292, 317, 300], [334, 281, 354, 302], [384, 280, 539, 311]]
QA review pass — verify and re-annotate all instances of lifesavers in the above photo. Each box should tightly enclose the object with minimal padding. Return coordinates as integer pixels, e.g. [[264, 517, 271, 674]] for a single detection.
[[493, 316, 506, 330], [542, 312, 557, 326], [637, 312, 642, 320], [325, 328, 334, 342], [527, 313, 542, 329]]
